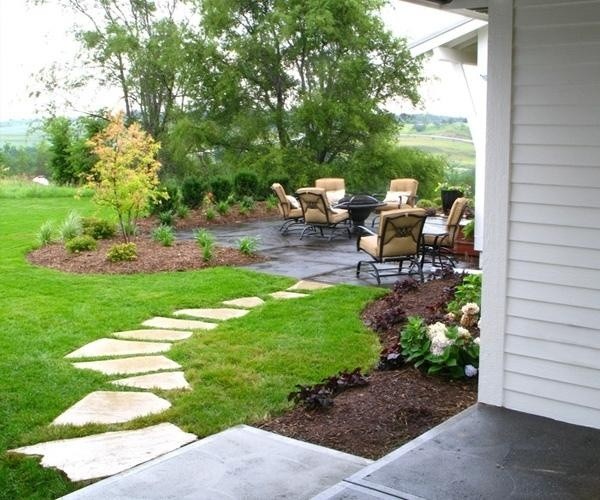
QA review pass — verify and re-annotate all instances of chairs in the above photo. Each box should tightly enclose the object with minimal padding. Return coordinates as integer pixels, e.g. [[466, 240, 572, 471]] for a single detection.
[[271, 175, 469, 286]]
